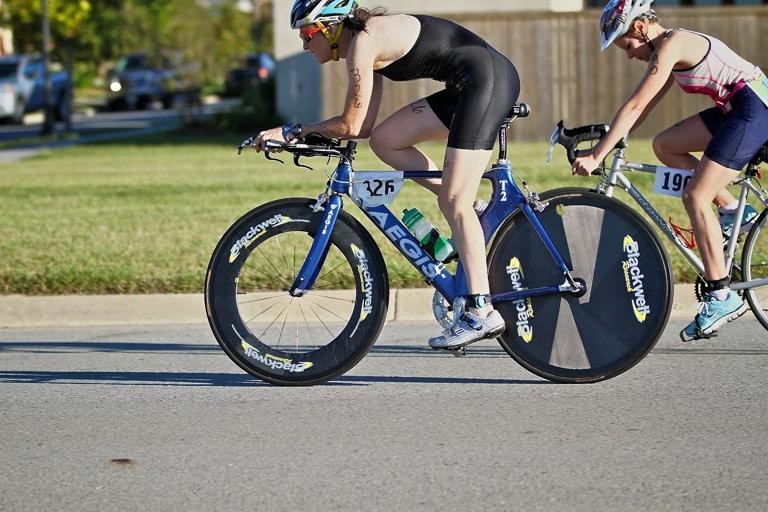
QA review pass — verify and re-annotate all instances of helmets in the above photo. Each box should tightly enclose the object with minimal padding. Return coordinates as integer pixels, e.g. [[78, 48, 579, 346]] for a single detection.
[[290, 0, 359, 30], [600, 0, 656, 53]]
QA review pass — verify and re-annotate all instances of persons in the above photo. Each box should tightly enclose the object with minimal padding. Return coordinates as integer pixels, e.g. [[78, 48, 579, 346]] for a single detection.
[[572, 0, 768, 338], [249, 0, 520, 349]]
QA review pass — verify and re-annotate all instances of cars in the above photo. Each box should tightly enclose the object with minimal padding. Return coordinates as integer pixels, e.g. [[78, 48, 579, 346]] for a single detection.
[[0, 49, 273, 123]]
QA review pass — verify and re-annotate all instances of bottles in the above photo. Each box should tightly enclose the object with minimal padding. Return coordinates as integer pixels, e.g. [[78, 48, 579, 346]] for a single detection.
[[400, 207, 454, 262]]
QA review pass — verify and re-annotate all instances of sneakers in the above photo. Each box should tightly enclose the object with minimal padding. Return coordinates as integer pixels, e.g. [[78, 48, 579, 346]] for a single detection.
[[442, 199, 490, 262], [428, 310, 507, 353], [685, 291, 748, 337], [718, 204, 760, 245]]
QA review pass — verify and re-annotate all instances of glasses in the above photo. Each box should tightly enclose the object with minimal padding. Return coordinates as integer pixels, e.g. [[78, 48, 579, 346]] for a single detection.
[[298, 25, 334, 43]]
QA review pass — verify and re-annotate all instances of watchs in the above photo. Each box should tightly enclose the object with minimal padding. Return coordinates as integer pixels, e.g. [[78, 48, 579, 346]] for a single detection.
[[282, 122, 303, 141]]
